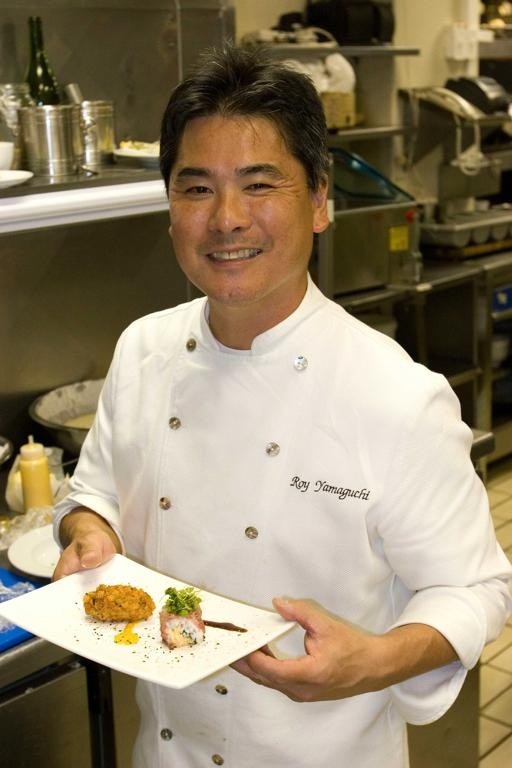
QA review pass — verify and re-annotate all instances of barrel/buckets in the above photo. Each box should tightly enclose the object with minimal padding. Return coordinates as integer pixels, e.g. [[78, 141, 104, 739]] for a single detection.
[[16, 104, 87, 176], [82, 100, 117, 164]]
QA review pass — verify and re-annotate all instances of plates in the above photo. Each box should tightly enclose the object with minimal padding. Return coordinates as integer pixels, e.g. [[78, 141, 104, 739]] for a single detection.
[[1, 139, 34, 190], [0, 553, 300, 692], [7, 523, 61, 579], [111, 151, 166, 170]]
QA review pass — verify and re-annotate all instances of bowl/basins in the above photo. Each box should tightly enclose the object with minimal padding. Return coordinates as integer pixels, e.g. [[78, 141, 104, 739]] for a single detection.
[[25, 376, 107, 459]]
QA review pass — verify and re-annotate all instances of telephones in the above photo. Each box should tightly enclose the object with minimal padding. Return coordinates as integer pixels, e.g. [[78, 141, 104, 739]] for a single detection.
[[431, 75, 511, 121]]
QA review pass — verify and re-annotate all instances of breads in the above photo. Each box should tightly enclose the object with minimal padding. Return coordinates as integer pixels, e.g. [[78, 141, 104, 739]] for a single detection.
[[82, 583, 155, 622]]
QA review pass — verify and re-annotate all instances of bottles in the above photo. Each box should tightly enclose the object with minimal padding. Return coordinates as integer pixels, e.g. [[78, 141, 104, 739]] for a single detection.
[[18, 434, 55, 514], [0, 14, 62, 138]]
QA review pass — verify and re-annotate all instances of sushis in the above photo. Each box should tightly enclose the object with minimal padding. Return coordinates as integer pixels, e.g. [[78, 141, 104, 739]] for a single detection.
[[159, 601, 205, 648]]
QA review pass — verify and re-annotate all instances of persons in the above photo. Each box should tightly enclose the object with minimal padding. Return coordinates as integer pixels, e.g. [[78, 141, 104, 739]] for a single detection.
[[53, 51, 512, 768]]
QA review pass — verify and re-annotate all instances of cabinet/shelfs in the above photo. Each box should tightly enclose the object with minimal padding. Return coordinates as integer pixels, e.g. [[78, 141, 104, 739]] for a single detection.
[[263, 43, 438, 221]]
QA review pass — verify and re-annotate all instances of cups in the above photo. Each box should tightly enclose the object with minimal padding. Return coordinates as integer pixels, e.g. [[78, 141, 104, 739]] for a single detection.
[[17, 96, 119, 175]]
